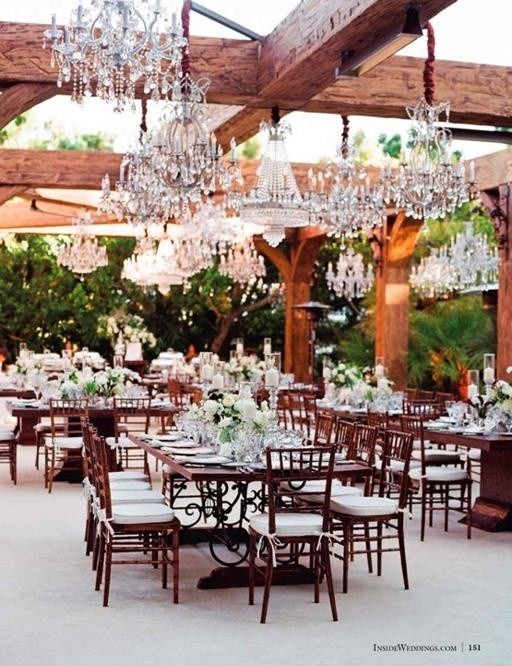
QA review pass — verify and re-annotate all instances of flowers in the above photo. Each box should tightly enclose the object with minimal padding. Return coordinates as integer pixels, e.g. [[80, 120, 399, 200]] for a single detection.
[[489, 379, 512, 419], [97, 314, 157, 348]]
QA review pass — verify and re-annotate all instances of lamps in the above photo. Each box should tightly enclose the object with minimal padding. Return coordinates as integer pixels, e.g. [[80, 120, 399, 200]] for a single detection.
[[43, 0, 502, 302]]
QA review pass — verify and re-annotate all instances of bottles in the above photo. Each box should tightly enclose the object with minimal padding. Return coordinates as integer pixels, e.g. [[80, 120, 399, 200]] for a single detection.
[[138, 433, 233, 464]]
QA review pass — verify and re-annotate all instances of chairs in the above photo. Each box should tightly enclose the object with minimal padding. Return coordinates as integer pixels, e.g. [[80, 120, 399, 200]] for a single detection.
[[0, 361, 474, 623]]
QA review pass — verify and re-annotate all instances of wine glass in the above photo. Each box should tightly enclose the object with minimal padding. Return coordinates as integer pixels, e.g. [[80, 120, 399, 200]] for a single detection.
[[34, 385, 40, 400], [446, 400, 512, 433], [174, 413, 215, 449]]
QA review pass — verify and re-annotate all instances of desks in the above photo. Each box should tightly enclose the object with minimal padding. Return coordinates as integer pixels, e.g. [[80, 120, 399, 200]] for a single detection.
[[389, 423, 512, 532]]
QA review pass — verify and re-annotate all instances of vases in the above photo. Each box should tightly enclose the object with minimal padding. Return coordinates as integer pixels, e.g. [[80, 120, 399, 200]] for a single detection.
[[125, 343, 144, 361]]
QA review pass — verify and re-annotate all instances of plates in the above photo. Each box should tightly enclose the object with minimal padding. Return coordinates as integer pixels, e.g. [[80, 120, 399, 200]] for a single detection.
[[289, 453, 346, 462], [414, 422, 453, 427]]
